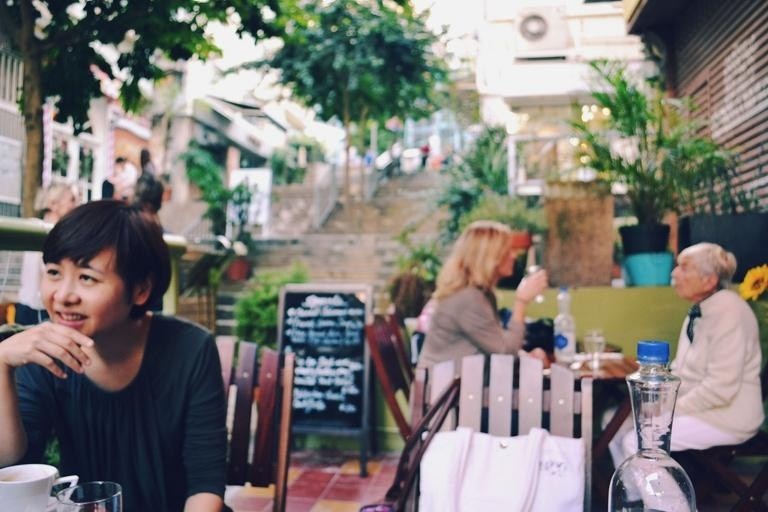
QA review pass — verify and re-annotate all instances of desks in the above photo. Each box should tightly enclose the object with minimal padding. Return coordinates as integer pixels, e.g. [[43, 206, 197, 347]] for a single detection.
[[512, 357, 676, 511]]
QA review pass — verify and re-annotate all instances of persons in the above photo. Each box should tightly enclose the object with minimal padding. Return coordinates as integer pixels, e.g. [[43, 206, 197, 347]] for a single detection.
[[407, 219, 548, 432], [134, 148, 164, 213], [0, 199, 235, 512], [601, 242, 766, 512], [106, 157, 137, 203]]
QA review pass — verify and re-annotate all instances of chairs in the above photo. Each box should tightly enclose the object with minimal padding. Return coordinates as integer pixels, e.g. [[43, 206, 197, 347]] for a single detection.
[[671, 367, 768, 512], [365, 316, 414, 441], [212, 334, 295, 511], [405, 352, 594, 512]]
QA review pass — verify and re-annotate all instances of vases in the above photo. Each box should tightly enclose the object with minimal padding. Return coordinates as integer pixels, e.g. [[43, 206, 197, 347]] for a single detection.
[[624, 251, 675, 287]]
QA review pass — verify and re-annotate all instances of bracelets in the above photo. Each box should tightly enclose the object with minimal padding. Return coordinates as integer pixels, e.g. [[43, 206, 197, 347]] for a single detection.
[[515, 293, 529, 311]]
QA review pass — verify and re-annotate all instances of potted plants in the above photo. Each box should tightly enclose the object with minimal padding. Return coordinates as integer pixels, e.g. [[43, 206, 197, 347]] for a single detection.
[[668, 93, 768, 284], [560, 52, 744, 252]]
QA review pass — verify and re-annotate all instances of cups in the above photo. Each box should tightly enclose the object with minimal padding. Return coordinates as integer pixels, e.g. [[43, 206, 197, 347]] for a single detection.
[[55, 480, 127, 512], [0, 462, 78, 511]]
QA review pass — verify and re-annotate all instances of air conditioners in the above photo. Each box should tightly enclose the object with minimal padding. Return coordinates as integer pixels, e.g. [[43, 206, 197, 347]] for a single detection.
[[512, 10, 564, 59]]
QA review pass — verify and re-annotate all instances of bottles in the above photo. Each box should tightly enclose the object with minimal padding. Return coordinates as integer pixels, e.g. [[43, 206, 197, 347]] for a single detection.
[[607, 337, 698, 511], [551, 287, 576, 364]]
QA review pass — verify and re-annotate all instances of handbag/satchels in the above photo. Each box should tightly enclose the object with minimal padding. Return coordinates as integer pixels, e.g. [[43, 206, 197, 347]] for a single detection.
[[417, 428, 586, 512]]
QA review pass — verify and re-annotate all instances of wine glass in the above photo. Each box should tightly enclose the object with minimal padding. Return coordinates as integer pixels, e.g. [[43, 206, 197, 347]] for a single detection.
[[583, 327, 607, 370]]
